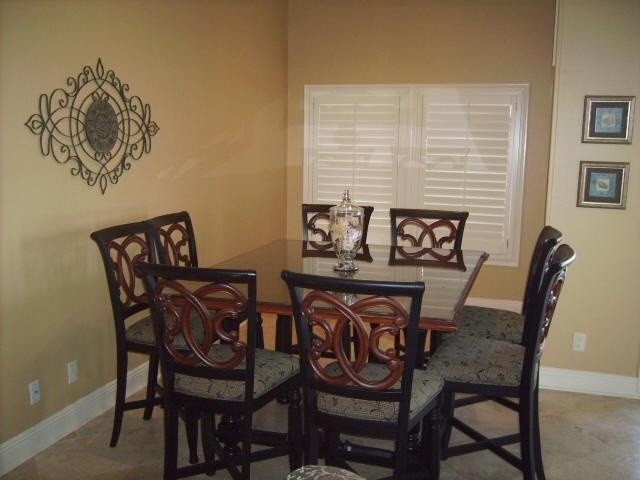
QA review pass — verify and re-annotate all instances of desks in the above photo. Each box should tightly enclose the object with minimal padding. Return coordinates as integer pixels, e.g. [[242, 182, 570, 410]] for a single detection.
[[137, 237, 490, 480]]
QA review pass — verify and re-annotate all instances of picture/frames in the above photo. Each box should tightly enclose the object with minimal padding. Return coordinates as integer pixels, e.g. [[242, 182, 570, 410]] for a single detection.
[[581, 94, 636, 144], [576, 160, 631, 209]]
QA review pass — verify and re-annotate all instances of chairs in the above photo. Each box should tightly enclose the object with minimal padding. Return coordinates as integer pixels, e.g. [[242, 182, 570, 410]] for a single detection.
[[273, 203, 374, 360], [430, 226, 563, 356], [426, 244, 575, 480], [279, 268, 444, 480], [91, 220, 230, 464], [139, 260, 303, 480], [149, 211, 267, 349], [374, 207, 469, 360]]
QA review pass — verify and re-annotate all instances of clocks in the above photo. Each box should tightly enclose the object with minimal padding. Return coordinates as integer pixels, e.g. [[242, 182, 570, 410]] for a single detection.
[[24, 58, 159, 196]]
